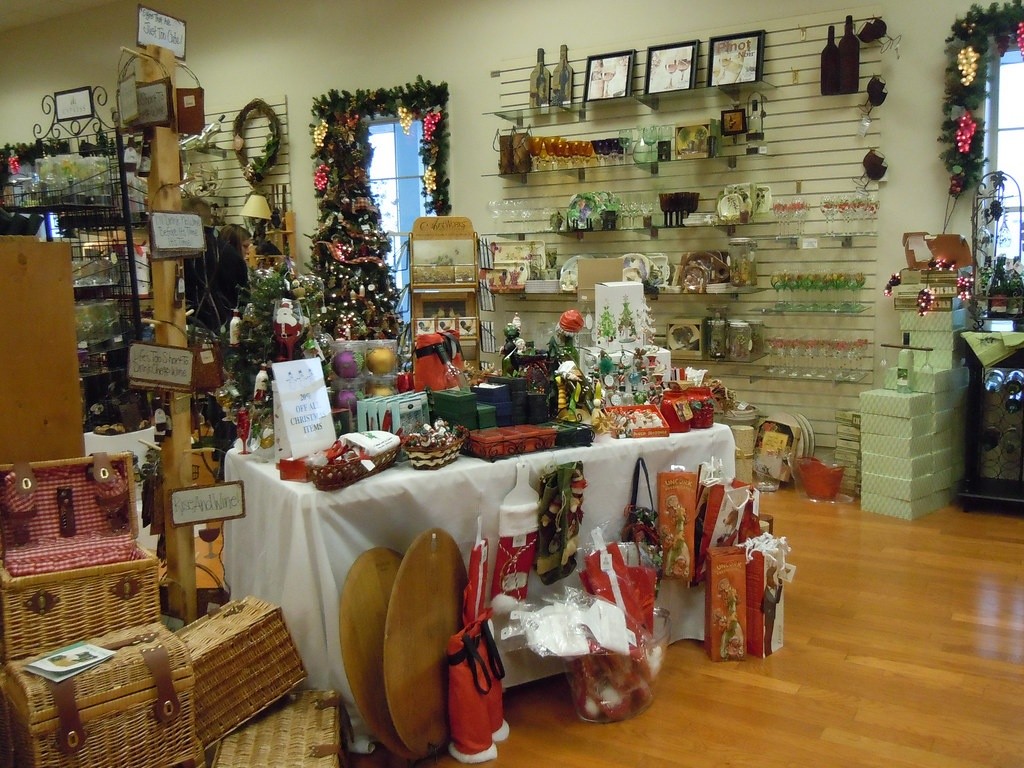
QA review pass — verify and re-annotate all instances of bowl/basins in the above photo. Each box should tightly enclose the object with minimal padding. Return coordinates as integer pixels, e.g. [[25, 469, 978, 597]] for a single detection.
[[559, 606, 670, 725]]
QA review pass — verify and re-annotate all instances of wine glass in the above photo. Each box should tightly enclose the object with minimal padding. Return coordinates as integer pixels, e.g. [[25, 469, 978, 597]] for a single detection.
[[658, 191, 699, 227], [766, 337, 868, 381], [878, 343, 891, 378], [919, 346, 933, 383], [616, 202, 652, 229], [528, 125, 673, 170]]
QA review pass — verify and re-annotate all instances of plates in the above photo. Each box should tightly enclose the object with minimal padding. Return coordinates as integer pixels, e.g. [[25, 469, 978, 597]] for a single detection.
[[524, 252, 650, 294]]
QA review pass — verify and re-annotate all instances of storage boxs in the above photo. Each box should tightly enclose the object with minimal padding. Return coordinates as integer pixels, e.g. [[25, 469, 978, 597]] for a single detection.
[[595, 282, 644, 351], [834, 307, 970, 520], [433, 379, 579, 459]]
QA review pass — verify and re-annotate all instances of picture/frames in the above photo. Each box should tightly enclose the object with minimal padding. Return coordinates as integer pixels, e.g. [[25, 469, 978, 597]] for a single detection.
[[643, 38, 700, 96], [583, 49, 636, 103], [722, 109, 747, 136], [706, 29, 766, 88]]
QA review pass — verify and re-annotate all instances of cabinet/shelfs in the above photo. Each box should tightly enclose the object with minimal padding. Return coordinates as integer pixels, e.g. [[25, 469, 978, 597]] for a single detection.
[[408, 215, 480, 373], [480, 79, 768, 363]]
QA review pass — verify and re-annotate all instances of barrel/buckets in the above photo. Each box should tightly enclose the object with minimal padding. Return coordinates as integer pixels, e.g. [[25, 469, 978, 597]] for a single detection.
[[797, 457, 846, 502]]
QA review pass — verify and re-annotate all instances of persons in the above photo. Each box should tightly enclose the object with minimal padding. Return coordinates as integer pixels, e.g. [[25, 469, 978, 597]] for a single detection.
[[183, 198, 252, 335]]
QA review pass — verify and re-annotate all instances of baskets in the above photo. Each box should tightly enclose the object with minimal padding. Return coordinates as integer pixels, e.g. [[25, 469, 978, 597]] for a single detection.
[[308, 439, 403, 492], [402, 425, 471, 470]]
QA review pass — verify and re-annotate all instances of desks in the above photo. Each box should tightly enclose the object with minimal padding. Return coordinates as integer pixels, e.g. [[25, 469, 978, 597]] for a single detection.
[[221, 420, 735, 753]]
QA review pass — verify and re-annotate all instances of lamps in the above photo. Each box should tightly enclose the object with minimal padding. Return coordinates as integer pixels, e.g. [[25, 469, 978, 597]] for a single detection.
[[238, 195, 272, 220]]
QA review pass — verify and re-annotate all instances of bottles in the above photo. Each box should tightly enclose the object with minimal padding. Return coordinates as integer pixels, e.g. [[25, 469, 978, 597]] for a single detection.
[[707, 318, 763, 360], [665, 517, 690, 577], [661, 390, 691, 433], [981, 255, 1024, 456], [820, 194, 879, 235], [770, 269, 866, 311], [729, 237, 758, 286], [895, 332, 914, 393], [687, 386, 714, 428], [719, 593, 743, 658], [773, 196, 810, 236], [558, 59, 570, 101], [435, 344, 471, 393], [536, 64, 546, 105]]
